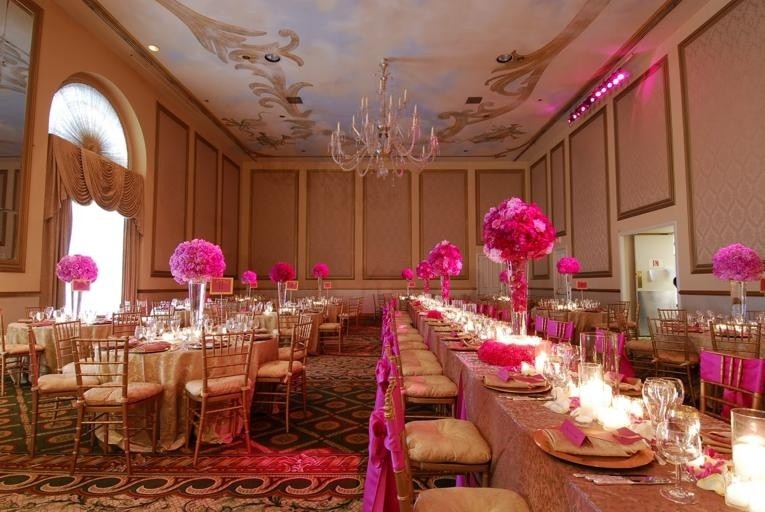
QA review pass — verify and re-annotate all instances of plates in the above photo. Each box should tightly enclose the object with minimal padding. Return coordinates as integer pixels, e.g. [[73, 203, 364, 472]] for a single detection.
[[17, 319, 33, 323]]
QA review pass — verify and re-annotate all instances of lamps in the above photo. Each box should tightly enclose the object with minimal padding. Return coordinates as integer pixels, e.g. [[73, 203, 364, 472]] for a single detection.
[[324, 55, 438, 185]]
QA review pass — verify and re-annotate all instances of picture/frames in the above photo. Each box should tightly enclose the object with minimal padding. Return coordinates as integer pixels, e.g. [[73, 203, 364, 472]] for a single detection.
[[248, 165, 531, 282], [0, 0, 46, 274], [149, 99, 244, 281]]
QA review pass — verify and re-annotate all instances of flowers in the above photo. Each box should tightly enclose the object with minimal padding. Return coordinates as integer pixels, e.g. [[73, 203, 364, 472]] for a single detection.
[[55, 254, 103, 285]]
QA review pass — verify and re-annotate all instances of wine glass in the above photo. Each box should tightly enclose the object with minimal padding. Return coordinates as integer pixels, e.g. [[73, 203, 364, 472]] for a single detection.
[[92, 295, 343, 353], [409, 292, 765, 512], [28, 306, 66, 325]]
